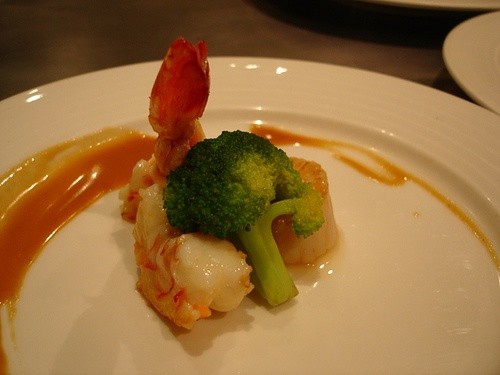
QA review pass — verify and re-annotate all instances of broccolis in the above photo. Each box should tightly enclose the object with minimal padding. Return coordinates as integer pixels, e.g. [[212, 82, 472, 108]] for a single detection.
[[166, 131, 325, 306]]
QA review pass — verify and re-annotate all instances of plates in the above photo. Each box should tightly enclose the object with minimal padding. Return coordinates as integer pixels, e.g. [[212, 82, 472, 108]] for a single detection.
[[0, 8, 497, 371]]
